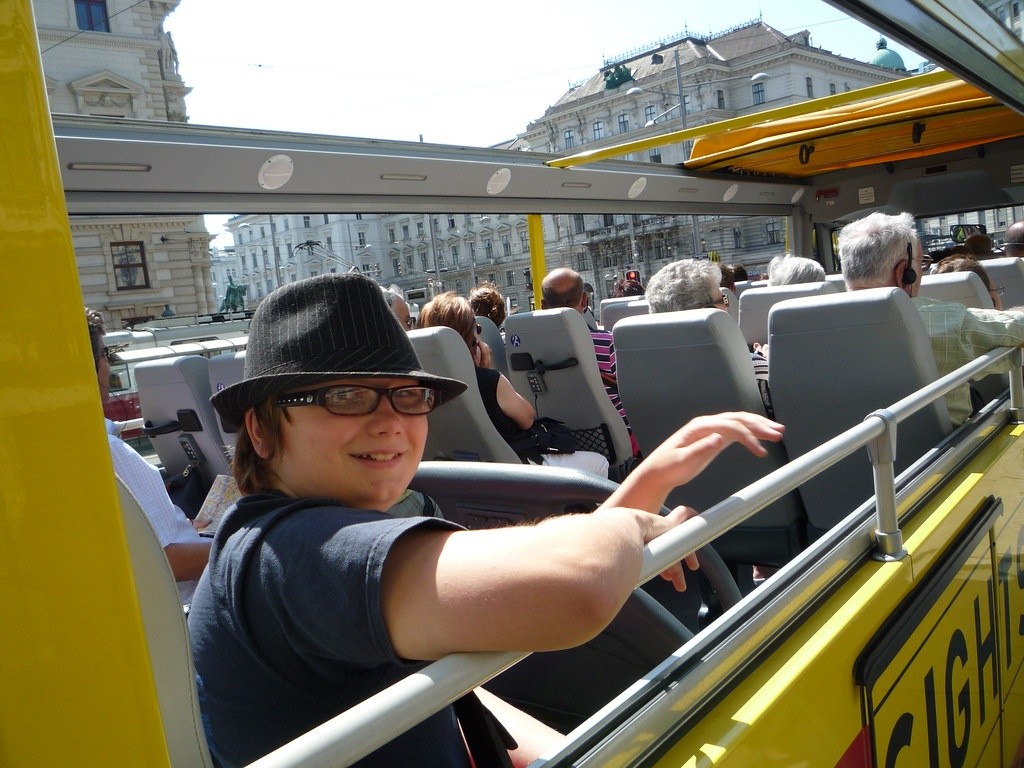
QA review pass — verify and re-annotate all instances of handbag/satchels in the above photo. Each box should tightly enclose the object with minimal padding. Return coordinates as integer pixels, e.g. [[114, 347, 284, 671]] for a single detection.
[[530, 417, 576, 455]]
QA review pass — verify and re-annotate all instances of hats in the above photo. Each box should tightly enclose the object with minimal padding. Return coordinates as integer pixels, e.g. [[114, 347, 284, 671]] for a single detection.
[[211, 273, 468, 424]]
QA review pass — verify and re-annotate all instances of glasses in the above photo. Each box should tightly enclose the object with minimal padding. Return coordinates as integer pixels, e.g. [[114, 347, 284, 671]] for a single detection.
[[474, 324, 482, 335], [95, 346, 111, 361], [710, 295, 730, 307], [997, 239, 1024, 249], [912, 254, 934, 272], [398, 315, 413, 329], [272, 384, 442, 417], [988, 284, 1005, 297]]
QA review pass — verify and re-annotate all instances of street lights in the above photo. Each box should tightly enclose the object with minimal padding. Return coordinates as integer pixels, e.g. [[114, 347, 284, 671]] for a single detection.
[[625, 73, 769, 259], [238, 223, 281, 287]]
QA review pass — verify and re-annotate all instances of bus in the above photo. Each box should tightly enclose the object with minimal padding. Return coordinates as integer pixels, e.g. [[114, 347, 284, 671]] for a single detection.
[[1, 1, 1024, 768]]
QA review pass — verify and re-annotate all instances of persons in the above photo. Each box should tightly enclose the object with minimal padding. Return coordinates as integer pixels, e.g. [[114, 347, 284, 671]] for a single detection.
[[839, 212, 1024, 431], [734, 265, 748, 282], [187, 273, 786, 768], [84, 308, 214, 606], [380, 253, 826, 479]]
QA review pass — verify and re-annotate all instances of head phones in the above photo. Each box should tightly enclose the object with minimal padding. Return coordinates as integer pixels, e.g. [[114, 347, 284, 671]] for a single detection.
[[902, 233, 917, 284]]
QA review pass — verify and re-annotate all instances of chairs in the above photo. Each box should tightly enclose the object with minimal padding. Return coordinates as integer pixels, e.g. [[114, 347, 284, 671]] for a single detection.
[[112, 256, 1023, 768]]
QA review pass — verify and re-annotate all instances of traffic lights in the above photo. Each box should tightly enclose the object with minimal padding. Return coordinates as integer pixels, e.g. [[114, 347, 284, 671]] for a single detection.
[[398, 261, 407, 276], [624, 271, 640, 285]]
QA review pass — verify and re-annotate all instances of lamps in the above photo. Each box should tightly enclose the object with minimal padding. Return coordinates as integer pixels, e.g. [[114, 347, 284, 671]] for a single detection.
[[161, 305, 176, 317]]
